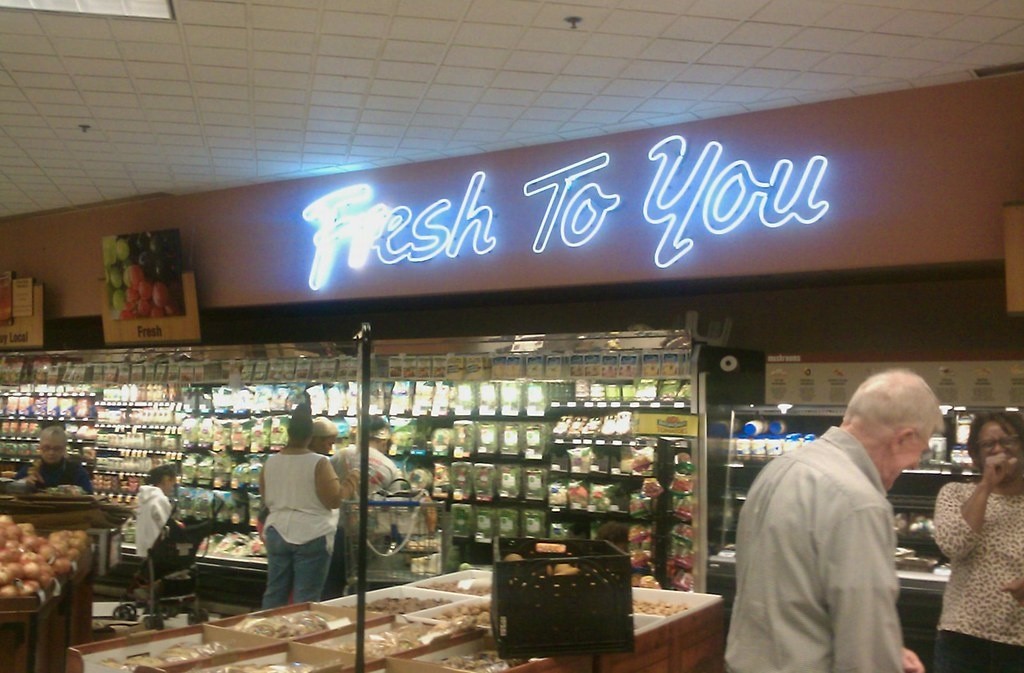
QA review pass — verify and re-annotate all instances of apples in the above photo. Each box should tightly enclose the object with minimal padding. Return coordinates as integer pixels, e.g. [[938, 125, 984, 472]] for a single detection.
[[0, 515, 87, 599]]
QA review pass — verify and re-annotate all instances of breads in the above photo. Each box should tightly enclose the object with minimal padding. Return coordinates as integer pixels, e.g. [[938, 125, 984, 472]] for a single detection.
[[90, 611, 534, 673]]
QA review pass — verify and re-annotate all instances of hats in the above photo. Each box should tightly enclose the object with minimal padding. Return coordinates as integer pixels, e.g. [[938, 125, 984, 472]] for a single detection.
[[312, 416, 339, 437]]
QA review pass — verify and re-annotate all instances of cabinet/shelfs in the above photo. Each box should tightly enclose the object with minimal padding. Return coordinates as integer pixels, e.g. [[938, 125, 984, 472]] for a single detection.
[[0, 328, 768, 593], [710, 404, 1024, 598]]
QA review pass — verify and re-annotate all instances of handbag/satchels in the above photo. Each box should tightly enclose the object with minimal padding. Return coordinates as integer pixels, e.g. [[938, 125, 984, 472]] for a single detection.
[[372, 478, 437, 535]]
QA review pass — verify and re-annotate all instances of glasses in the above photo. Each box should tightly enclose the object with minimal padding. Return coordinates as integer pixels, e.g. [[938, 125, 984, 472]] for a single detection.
[[39, 444, 66, 453], [915, 429, 933, 466], [975, 434, 1021, 455]]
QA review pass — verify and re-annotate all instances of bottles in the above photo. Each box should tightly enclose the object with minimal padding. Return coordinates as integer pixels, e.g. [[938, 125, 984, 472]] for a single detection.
[[735, 420, 814, 462]]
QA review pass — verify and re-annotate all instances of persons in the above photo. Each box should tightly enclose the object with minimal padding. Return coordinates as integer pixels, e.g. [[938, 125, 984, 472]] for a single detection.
[[720, 366, 941, 673], [5, 425, 96, 496], [256, 409, 402, 610], [133, 464, 215, 565], [932, 409, 1024, 673]]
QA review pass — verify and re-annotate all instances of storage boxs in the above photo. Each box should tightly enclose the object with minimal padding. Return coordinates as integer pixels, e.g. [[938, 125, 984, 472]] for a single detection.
[[76, 567, 723, 673], [487, 534, 636, 659]]
